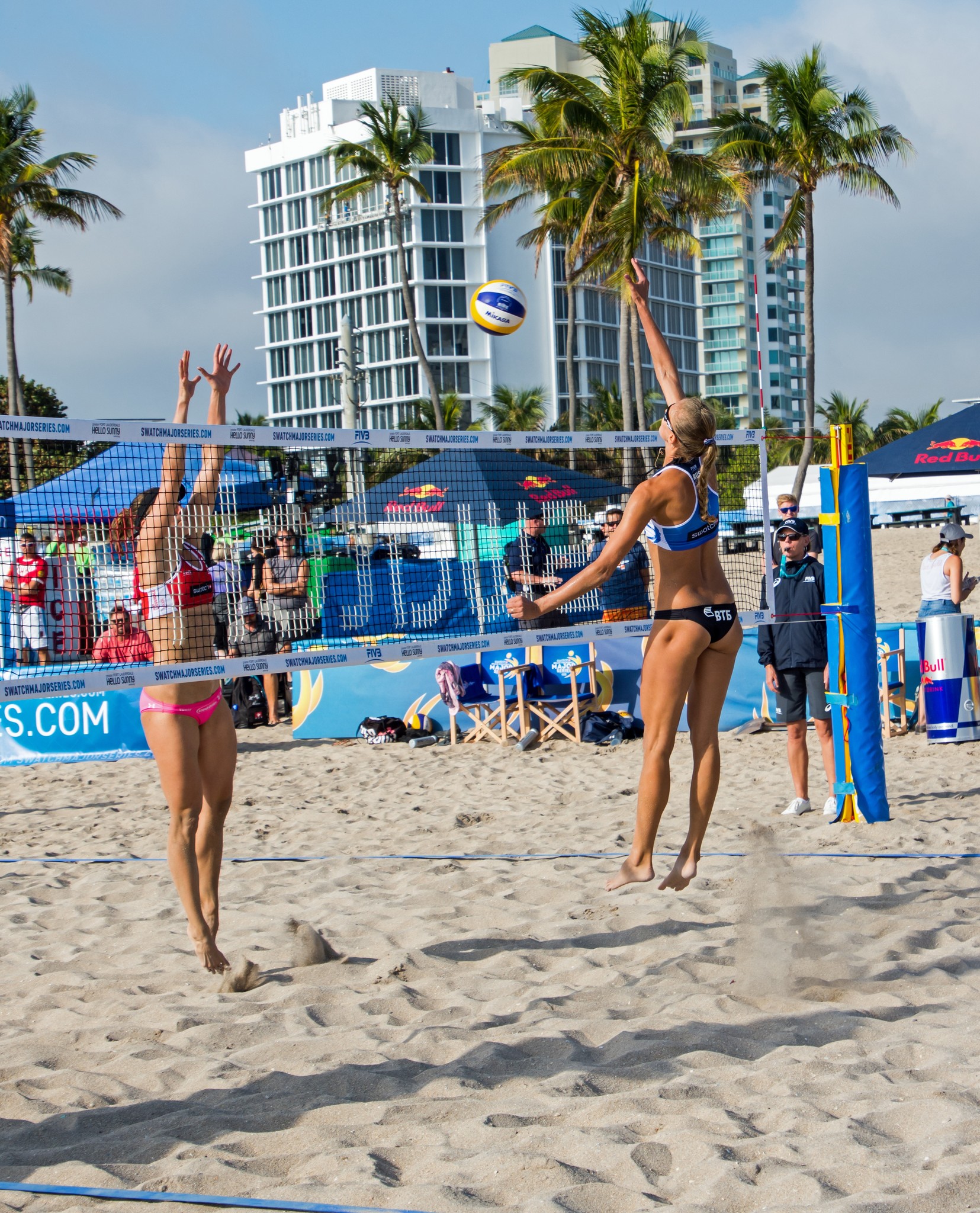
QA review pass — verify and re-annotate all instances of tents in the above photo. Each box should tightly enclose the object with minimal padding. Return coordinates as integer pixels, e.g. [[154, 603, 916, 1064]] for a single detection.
[[0, 441, 633, 635]]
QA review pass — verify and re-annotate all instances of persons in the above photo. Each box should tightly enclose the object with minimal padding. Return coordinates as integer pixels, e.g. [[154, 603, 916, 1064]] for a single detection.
[[942, 496, 954, 517], [2, 526, 319, 726], [107, 343, 242, 975], [915, 523, 979, 734], [757, 518, 836, 816], [505, 513, 564, 632], [590, 508, 650, 625], [506, 258, 744, 892], [761, 493, 822, 575]]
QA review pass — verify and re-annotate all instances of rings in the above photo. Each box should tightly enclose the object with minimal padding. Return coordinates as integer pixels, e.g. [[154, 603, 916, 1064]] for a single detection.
[[511, 608, 514, 613]]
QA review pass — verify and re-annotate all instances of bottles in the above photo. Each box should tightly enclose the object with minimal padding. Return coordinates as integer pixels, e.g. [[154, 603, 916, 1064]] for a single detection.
[[409, 735, 440, 748], [611, 730, 622, 745], [501, 738, 518, 747], [514, 728, 539, 751]]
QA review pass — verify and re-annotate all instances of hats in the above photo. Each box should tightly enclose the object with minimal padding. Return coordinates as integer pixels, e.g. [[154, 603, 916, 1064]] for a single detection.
[[237, 596, 256, 616], [128, 483, 186, 541], [776, 517, 809, 534], [939, 522, 974, 542], [77, 536, 87, 544], [945, 495, 954, 500], [55, 531, 65, 538]]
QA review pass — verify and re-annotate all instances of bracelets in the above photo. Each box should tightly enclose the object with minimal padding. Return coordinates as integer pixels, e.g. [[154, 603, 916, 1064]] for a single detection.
[[963, 588, 971, 592]]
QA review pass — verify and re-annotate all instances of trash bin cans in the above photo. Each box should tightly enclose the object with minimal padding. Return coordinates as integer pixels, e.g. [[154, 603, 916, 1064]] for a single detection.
[[915, 612, 980, 743]]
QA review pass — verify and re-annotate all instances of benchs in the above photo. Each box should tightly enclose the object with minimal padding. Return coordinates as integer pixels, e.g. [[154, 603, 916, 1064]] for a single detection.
[[880, 513, 974, 529], [721, 523, 881, 556]]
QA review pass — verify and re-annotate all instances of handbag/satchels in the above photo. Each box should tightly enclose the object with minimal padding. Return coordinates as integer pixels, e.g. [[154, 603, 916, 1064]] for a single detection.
[[356, 715, 406, 744], [580, 710, 645, 742], [211, 592, 236, 625]]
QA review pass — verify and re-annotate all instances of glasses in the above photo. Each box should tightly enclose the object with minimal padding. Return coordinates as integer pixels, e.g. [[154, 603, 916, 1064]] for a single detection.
[[110, 618, 129, 624], [240, 614, 254, 619], [777, 533, 808, 541], [779, 506, 798, 514], [664, 403, 692, 453], [19, 543, 35, 547], [276, 535, 294, 541], [535, 516, 548, 524], [606, 520, 620, 527]]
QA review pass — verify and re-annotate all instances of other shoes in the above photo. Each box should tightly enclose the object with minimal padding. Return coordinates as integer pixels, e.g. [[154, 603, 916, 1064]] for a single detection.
[[780, 798, 811, 816], [823, 796, 837, 815], [914, 724, 926, 735]]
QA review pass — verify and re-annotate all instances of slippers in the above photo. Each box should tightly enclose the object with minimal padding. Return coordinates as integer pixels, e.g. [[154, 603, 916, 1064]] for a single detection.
[[435, 733, 470, 746]]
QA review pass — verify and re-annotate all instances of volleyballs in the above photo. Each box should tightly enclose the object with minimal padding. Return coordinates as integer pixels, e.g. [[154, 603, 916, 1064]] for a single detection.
[[470, 279, 528, 337]]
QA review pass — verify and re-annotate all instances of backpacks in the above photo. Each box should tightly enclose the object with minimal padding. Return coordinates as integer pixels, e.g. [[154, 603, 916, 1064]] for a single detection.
[[232, 676, 269, 729]]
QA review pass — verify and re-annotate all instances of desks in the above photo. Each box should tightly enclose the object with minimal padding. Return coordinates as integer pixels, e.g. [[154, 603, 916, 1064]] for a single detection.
[[885, 505, 966, 526], [726, 514, 878, 549]]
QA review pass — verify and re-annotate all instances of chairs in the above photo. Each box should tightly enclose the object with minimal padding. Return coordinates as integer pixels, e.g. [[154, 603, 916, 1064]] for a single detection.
[[450, 647, 530, 744], [515, 642, 597, 742], [875, 629, 908, 738]]
